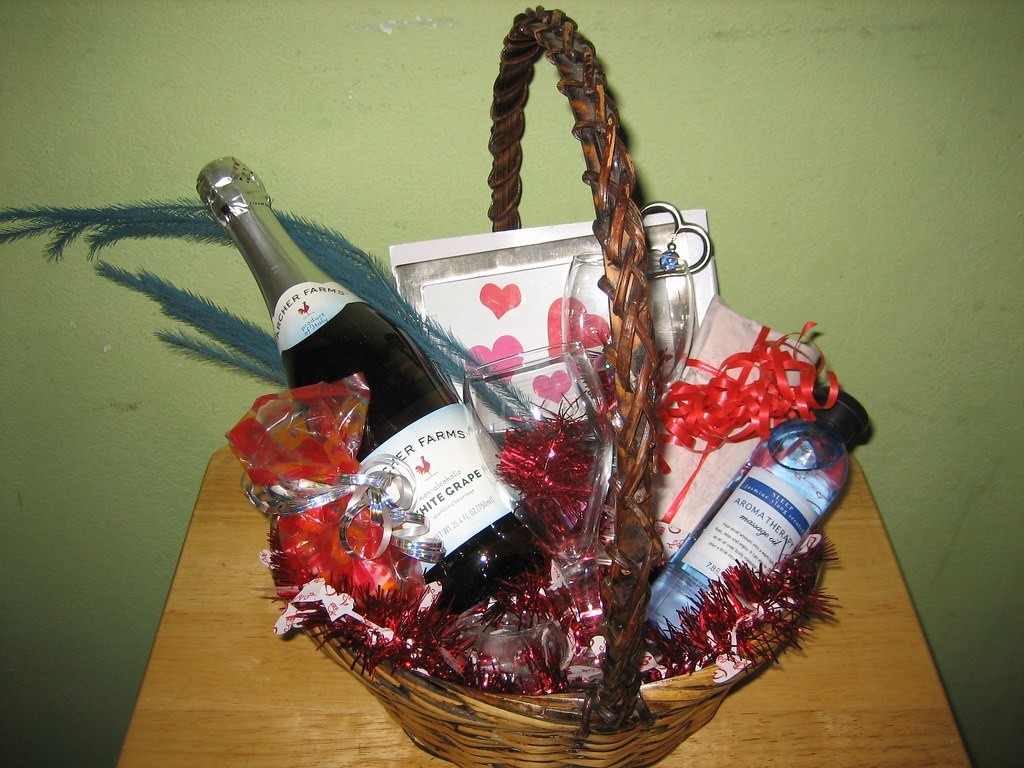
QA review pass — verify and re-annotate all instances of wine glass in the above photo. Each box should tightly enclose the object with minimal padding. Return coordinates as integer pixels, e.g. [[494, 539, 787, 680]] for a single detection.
[[551, 251, 696, 568], [463, 340, 615, 667]]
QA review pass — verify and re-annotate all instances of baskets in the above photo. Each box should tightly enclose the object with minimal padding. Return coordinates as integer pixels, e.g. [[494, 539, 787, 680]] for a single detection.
[[263, 6, 827, 768]]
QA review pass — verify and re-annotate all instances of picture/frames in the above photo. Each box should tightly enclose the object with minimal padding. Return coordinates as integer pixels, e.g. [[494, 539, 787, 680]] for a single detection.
[[397, 223, 702, 419]]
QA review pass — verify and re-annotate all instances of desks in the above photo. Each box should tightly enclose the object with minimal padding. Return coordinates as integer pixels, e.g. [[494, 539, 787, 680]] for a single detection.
[[106, 441, 972, 768]]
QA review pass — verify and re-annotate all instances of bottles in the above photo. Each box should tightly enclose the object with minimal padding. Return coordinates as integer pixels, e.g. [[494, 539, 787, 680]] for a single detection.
[[195, 156, 541, 615], [646, 387, 872, 657]]
